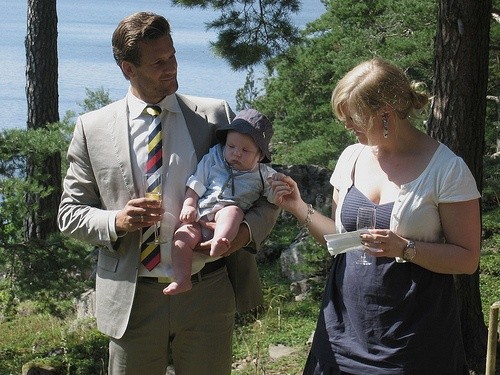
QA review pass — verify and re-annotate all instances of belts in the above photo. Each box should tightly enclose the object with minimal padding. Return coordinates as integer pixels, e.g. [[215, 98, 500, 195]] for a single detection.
[[138, 256, 226, 284]]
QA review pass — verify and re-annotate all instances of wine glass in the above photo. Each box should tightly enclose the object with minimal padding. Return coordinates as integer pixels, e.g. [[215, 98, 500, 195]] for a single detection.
[[143, 173, 168, 246], [354, 207, 376, 266]]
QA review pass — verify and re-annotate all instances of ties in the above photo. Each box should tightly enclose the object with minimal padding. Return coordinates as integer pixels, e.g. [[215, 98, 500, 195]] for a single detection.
[[140, 105, 163, 272]]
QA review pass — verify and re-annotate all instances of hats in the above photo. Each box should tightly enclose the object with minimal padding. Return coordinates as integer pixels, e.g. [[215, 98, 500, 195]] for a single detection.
[[210, 109, 274, 164]]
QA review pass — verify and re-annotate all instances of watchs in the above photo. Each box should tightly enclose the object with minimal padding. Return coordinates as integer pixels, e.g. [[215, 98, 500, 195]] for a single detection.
[[402, 240, 417, 262]]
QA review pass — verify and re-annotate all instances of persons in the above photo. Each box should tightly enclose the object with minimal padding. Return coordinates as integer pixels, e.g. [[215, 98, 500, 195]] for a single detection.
[[54, 10, 286, 375], [162, 108, 287, 296], [266, 58, 482, 375]]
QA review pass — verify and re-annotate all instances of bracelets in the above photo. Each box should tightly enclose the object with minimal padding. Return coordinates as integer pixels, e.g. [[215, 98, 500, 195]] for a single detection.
[[297, 203, 315, 234]]
